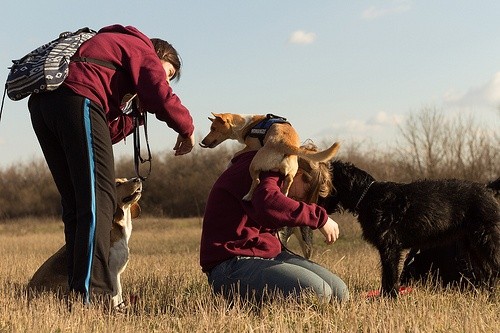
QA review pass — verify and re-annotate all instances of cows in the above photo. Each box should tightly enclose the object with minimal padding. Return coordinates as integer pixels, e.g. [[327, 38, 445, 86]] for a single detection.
[[270, 143, 338, 261]]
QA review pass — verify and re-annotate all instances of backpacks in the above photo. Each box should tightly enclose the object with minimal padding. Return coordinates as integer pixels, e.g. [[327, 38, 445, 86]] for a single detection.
[[5, 27, 130, 101]]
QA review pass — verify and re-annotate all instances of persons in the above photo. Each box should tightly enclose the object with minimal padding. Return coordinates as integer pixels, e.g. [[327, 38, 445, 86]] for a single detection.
[[199, 128, 351, 305], [22, 23, 195, 311]]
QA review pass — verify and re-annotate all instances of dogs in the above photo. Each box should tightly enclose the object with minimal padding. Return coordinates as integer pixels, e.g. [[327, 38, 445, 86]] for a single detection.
[[198, 112, 340, 203], [316, 160, 500, 301], [25, 176, 143, 312]]
